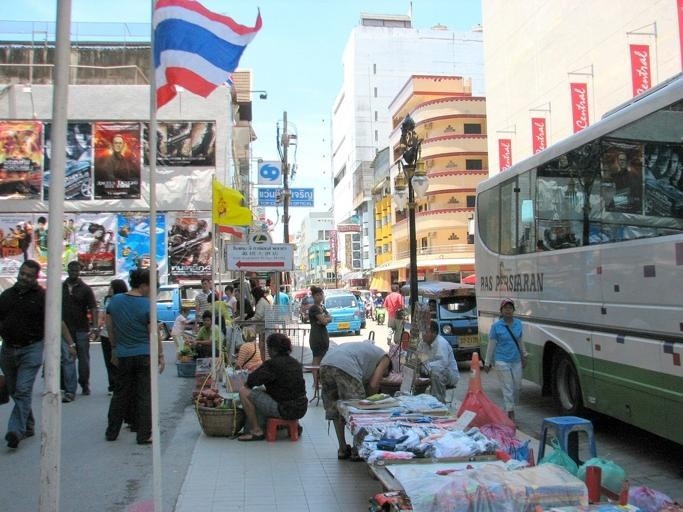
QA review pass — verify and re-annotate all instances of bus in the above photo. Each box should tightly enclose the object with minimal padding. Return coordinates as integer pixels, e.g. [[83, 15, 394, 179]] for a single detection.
[[474, 73, 683, 444]]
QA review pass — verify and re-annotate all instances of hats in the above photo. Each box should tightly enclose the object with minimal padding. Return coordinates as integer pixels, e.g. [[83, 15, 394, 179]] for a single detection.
[[501, 298, 516, 307]]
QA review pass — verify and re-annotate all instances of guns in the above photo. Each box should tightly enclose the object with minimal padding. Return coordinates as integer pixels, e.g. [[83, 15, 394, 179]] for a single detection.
[[8, 227, 13, 232]]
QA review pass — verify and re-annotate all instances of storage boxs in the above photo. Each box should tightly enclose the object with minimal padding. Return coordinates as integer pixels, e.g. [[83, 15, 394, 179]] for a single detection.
[[174, 360, 196, 377]]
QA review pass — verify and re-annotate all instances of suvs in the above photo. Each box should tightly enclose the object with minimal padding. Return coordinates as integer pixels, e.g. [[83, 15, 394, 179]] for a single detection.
[[300, 296, 315, 323]]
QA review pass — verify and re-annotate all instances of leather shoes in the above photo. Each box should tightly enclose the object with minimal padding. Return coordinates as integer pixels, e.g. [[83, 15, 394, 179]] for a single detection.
[[138, 436, 152, 444], [20, 430, 37, 437], [81, 384, 91, 394], [62, 393, 75, 402], [106, 432, 118, 441], [6, 432, 18, 449]]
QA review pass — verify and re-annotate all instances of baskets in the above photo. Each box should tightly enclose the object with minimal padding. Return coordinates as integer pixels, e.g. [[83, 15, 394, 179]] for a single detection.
[[194, 354, 248, 437]]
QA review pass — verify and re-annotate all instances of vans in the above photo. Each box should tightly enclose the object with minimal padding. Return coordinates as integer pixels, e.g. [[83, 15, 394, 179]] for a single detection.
[[156, 279, 255, 341], [322, 290, 366, 336], [402, 281, 492, 368]]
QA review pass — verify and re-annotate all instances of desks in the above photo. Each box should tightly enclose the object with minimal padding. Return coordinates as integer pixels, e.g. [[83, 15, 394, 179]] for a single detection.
[[305, 366, 321, 406]]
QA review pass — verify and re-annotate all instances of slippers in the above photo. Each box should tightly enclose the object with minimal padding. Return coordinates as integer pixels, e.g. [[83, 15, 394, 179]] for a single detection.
[[239, 432, 266, 441], [338, 443, 351, 458], [351, 449, 365, 460]]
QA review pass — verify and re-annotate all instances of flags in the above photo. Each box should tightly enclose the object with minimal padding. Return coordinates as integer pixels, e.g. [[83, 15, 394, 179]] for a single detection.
[[218, 226, 244, 239], [153, 0, 261, 115], [211, 174, 251, 225]]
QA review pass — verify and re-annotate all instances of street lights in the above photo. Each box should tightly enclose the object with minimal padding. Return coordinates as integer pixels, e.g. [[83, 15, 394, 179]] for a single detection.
[[392, 113, 429, 322]]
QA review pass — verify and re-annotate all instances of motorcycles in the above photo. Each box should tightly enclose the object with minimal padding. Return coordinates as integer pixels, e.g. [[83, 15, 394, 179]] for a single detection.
[[375, 302, 386, 325]]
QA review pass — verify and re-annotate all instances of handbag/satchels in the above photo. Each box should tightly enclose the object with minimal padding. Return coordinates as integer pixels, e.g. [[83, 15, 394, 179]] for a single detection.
[[505, 324, 529, 367], [390, 294, 404, 319]]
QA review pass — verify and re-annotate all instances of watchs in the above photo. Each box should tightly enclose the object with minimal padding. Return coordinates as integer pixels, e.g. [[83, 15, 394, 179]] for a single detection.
[[69, 343, 76, 349]]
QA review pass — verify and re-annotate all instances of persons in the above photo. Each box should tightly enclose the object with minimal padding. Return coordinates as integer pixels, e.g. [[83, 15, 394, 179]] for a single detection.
[[0, 260, 77, 448], [415, 321, 459, 404], [97, 134, 137, 192], [383, 285, 404, 345], [245, 287, 270, 361], [611, 151, 640, 211], [232, 288, 253, 319], [363, 295, 369, 318], [60, 261, 98, 402], [184, 310, 224, 358], [207, 293, 231, 347], [100, 279, 128, 395], [13, 226, 28, 262], [222, 285, 236, 318], [320, 342, 393, 461], [105, 269, 165, 442], [68, 219, 75, 234], [239, 333, 306, 440], [63, 221, 72, 241], [170, 308, 194, 365], [271, 286, 290, 321], [34, 217, 46, 252], [308, 286, 331, 388], [373, 293, 384, 309], [234, 327, 262, 372], [484, 299, 528, 426], [194, 279, 211, 328], [263, 289, 272, 306], [641, 145, 682, 189], [536, 219, 581, 253]]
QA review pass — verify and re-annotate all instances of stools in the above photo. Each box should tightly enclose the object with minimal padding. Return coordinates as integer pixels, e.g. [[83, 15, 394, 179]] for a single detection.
[[430, 385, 456, 408], [266, 418, 298, 442], [537, 417, 597, 469]]
